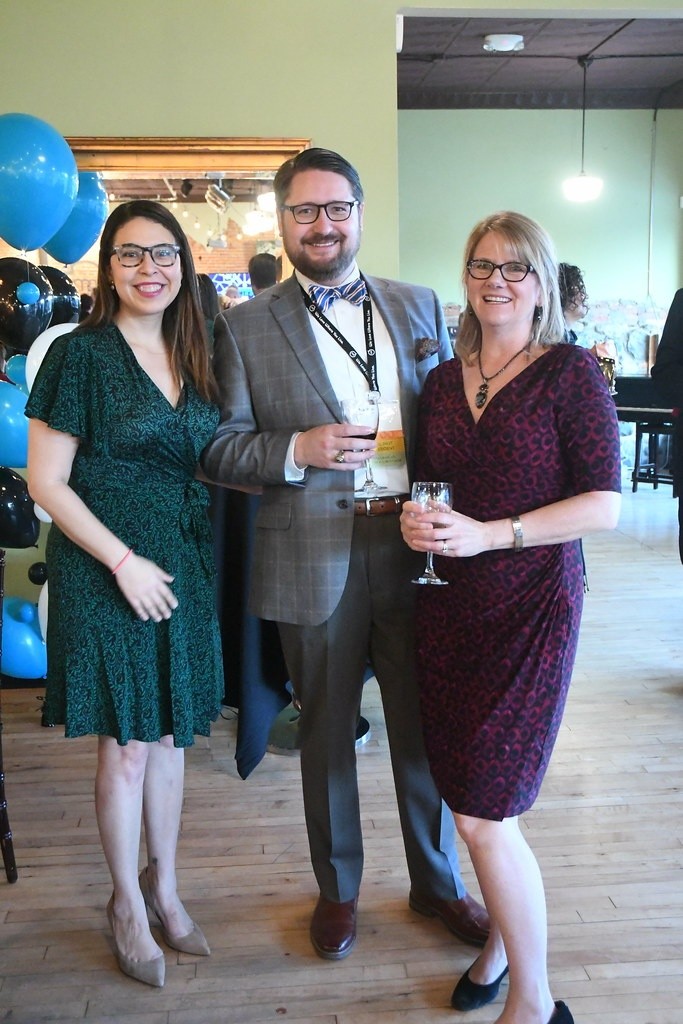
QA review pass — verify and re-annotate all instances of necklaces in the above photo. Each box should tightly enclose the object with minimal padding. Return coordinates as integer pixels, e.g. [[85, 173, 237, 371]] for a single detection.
[[475, 338, 537, 409]]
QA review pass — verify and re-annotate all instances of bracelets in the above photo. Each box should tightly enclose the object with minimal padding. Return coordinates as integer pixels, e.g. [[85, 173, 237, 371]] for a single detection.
[[112, 549, 133, 574], [510, 515, 524, 552]]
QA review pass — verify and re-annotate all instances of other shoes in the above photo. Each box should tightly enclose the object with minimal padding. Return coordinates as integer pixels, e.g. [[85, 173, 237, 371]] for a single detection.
[[450, 953, 510, 1011], [545, 999, 575, 1024]]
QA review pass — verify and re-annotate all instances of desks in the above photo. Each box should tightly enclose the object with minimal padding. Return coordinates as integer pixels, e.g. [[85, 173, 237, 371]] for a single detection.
[[616, 407, 675, 494]]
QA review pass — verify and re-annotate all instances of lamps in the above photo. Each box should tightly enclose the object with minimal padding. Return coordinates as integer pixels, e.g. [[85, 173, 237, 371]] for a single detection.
[[204, 178, 235, 215], [562, 58, 604, 201], [181, 180, 193, 199]]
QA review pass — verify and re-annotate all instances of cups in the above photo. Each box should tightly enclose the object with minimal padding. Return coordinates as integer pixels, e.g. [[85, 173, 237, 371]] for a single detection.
[[594, 338, 619, 396]]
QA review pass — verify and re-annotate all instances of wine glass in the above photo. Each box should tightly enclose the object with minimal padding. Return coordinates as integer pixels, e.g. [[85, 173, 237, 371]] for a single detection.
[[411, 481, 453, 584], [341, 392, 389, 496]]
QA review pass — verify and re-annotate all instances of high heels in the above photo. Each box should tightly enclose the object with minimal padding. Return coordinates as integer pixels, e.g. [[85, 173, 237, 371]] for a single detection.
[[137, 866, 211, 958], [106, 888, 166, 988]]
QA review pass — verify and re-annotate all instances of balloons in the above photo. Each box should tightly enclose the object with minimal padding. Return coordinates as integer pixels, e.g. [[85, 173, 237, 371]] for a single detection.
[[0, 257, 53, 354], [0, 114, 79, 251], [38, 265, 81, 328], [0, 325, 81, 550], [42, 171, 109, 264], [0, 562, 49, 679]]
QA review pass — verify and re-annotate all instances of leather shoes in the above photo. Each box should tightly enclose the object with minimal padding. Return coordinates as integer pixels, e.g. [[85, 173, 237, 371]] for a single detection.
[[310, 887, 359, 960], [408, 883, 491, 947]]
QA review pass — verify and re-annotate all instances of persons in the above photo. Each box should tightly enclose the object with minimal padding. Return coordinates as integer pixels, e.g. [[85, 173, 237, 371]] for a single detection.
[[651, 287, 683, 564], [198, 147, 489, 959], [25, 200, 225, 988], [79, 288, 96, 323], [197, 254, 277, 317], [399, 214, 622, 1024], [558, 261, 589, 344]]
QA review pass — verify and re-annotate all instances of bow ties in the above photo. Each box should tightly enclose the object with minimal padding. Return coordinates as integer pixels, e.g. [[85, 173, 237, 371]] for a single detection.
[[308, 278, 366, 313]]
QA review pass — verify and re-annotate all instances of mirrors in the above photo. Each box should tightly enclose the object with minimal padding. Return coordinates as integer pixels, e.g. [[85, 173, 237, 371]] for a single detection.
[[68, 136, 311, 305]]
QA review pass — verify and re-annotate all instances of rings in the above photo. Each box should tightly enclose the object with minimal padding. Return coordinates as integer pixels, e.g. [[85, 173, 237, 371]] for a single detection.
[[443, 541, 448, 553], [336, 450, 346, 464]]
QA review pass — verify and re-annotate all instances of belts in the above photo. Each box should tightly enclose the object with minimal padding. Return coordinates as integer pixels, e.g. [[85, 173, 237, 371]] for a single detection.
[[352, 492, 411, 517]]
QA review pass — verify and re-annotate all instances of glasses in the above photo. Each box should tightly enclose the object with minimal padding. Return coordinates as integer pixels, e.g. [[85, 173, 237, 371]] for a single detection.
[[107, 243, 182, 267], [280, 200, 361, 225], [465, 259, 537, 282]]
